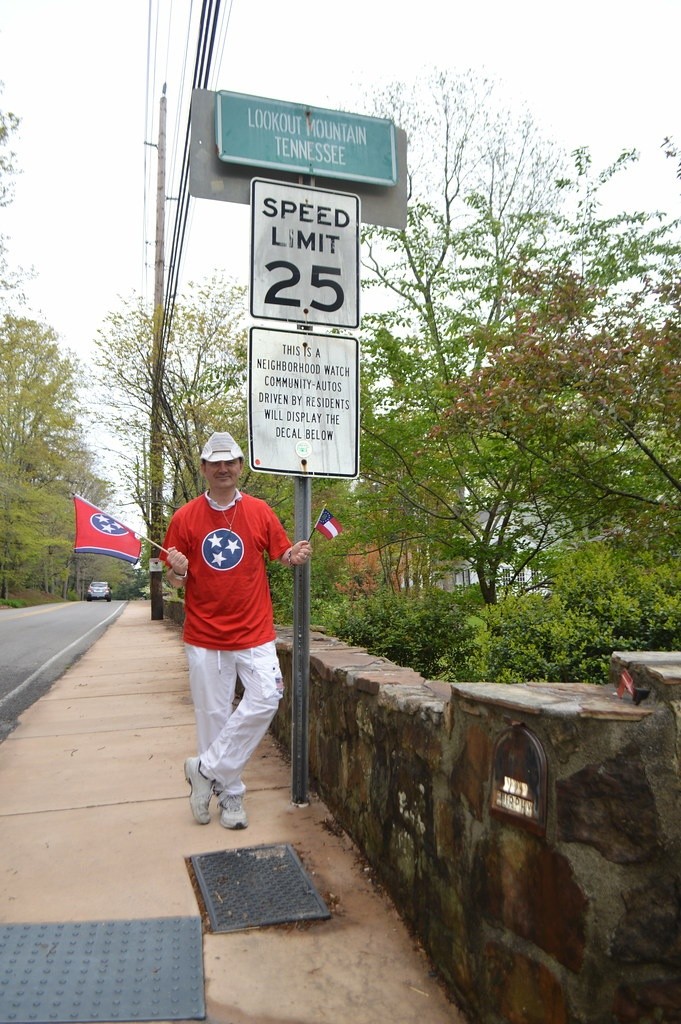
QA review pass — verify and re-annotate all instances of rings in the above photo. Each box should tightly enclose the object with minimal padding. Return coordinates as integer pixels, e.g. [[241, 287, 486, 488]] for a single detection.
[[304, 555, 307, 560]]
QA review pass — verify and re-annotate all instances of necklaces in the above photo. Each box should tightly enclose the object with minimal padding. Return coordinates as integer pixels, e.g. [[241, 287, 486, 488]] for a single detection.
[[222, 501, 238, 529]]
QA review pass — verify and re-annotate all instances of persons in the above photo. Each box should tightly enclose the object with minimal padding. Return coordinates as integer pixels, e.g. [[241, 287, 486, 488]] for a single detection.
[[159, 432, 313, 831]]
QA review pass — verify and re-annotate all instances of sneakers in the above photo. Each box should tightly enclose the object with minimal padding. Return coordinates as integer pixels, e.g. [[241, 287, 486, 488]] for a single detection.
[[217, 793, 248, 829], [184, 757, 216, 824]]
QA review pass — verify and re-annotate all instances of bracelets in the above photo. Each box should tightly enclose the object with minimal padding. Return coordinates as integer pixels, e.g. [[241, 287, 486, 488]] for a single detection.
[[287, 549, 296, 568], [170, 569, 187, 580]]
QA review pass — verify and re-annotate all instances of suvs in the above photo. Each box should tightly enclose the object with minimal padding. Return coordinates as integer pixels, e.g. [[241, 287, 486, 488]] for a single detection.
[[87, 582, 112, 603]]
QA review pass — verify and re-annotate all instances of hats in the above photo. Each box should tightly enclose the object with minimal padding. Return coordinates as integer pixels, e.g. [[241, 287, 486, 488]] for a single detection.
[[200, 432, 245, 465]]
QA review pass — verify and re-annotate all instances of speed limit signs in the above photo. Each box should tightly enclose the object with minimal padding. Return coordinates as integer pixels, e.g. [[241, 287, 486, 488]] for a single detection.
[[249, 175, 361, 332]]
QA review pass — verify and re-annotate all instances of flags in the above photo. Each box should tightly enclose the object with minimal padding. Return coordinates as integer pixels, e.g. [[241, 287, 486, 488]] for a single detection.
[[72, 497, 141, 565], [315, 508, 343, 541]]
